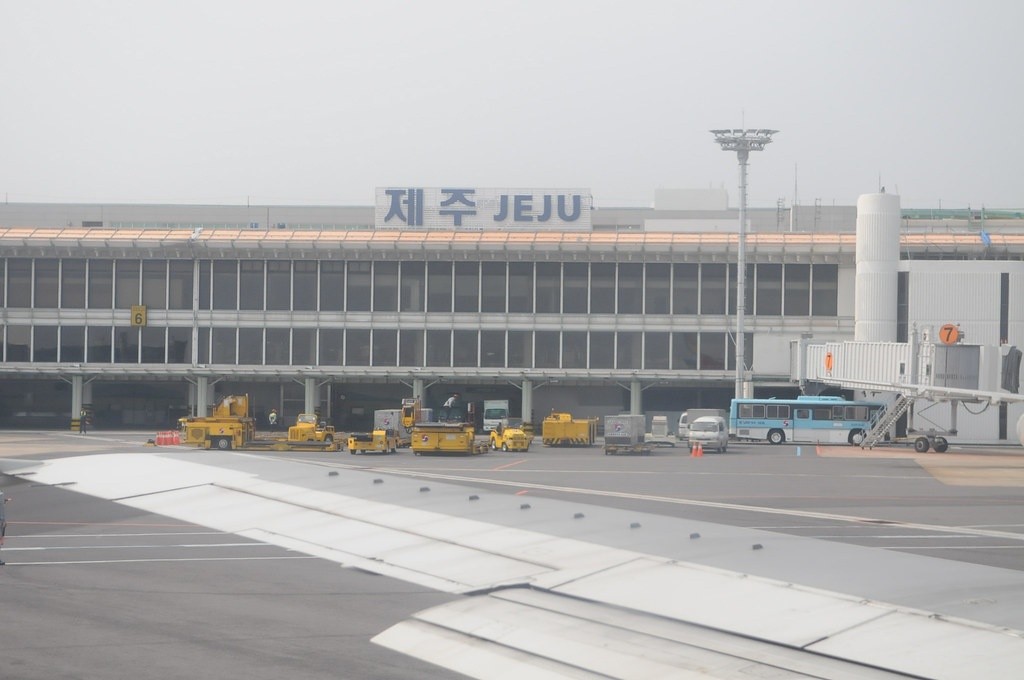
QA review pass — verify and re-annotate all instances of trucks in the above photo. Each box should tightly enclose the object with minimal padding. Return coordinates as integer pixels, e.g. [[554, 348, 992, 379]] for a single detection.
[[483, 399, 509, 434]]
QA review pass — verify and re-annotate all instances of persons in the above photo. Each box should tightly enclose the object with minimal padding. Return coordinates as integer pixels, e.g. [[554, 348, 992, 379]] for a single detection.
[[496, 423, 503, 435], [269, 408, 277, 431], [442, 393, 460, 421], [79, 407, 87, 436]]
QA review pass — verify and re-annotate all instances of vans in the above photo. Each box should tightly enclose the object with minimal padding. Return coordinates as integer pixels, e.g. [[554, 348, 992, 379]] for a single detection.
[[678, 410, 688, 441], [688, 415, 728, 453]]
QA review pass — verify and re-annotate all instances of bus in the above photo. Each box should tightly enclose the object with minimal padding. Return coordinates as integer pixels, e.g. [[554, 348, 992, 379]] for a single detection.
[[729, 394, 888, 446]]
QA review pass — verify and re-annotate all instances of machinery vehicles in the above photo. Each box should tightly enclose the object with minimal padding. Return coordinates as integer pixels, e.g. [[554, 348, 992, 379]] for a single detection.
[[178, 393, 346, 451], [402, 396, 489, 454], [489, 426, 530, 451], [348, 425, 399, 454], [287, 414, 335, 442]]
